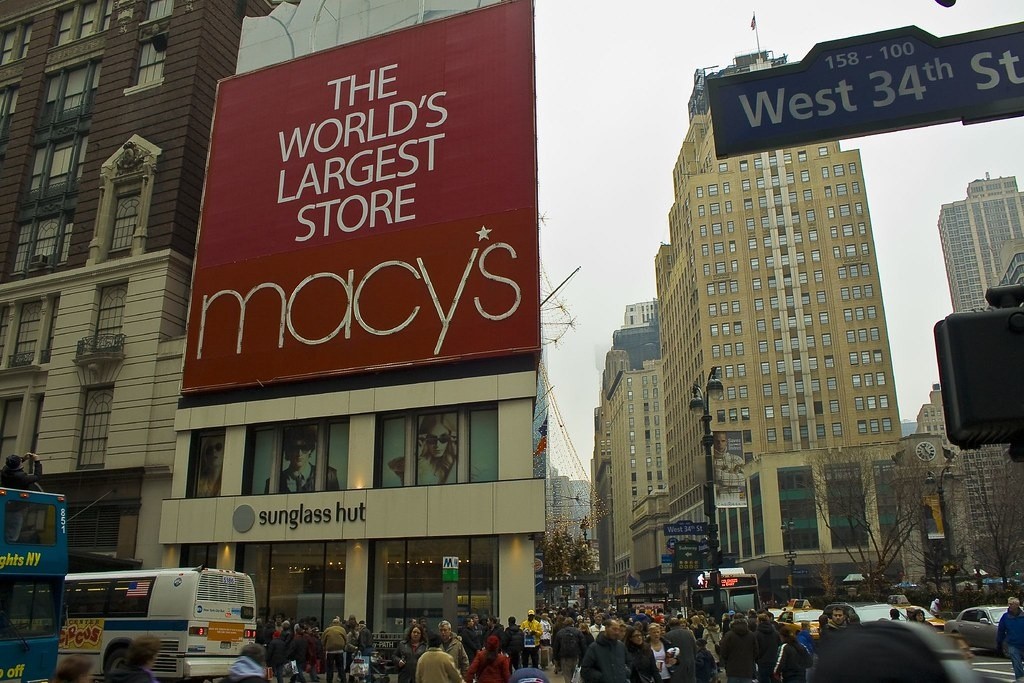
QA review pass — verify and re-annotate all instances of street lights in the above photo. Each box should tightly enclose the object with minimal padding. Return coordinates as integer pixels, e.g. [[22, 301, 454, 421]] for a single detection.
[[687, 365, 724, 566], [924, 464, 959, 590]]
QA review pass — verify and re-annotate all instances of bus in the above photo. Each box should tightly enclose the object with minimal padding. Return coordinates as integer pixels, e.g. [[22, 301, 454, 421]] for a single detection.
[[0, 487, 70, 683], [678, 568, 761, 617], [56, 564, 257, 683]]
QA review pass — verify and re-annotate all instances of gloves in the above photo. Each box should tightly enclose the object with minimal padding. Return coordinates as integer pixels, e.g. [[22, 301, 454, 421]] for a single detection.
[[997, 643, 1003, 651]]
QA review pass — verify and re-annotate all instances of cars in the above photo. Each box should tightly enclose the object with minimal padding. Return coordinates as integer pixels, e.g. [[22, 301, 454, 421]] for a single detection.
[[891, 567, 1024, 593], [943, 605, 1024, 659], [767, 598, 947, 646]]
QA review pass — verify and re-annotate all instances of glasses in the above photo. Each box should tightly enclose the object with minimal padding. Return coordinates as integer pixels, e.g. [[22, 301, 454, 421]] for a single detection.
[[412, 631, 422, 634]]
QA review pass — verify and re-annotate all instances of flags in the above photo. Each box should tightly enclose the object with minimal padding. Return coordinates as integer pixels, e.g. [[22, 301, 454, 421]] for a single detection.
[[751, 16, 755, 30]]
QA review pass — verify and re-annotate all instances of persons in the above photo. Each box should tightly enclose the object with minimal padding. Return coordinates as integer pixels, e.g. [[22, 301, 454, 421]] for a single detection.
[[0, 452, 43, 490], [713, 431, 745, 494], [995, 597, 1024, 680], [195, 435, 226, 497], [219, 602, 975, 683], [388, 413, 457, 485], [102, 634, 159, 683], [54, 655, 94, 683], [264, 425, 339, 494], [930, 598, 940, 618]]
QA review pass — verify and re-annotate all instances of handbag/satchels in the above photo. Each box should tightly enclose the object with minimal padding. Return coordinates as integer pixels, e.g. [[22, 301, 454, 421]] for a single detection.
[[524, 634, 536, 647], [306, 660, 312, 672], [715, 644, 721, 654], [268, 667, 273, 680], [354, 651, 364, 662], [291, 660, 299, 674], [350, 651, 369, 675], [541, 639, 551, 646], [639, 673, 654, 683], [571, 659, 584, 683]]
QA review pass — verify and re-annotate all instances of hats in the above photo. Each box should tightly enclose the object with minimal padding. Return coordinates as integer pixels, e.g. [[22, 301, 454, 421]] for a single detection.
[[296, 628, 303, 635], [527, 608, 535, 616], [578, 623, 588, 632], [486, 635, 499, 651], [6, 455, 20, 469], [273, 631, 281, 639]]
